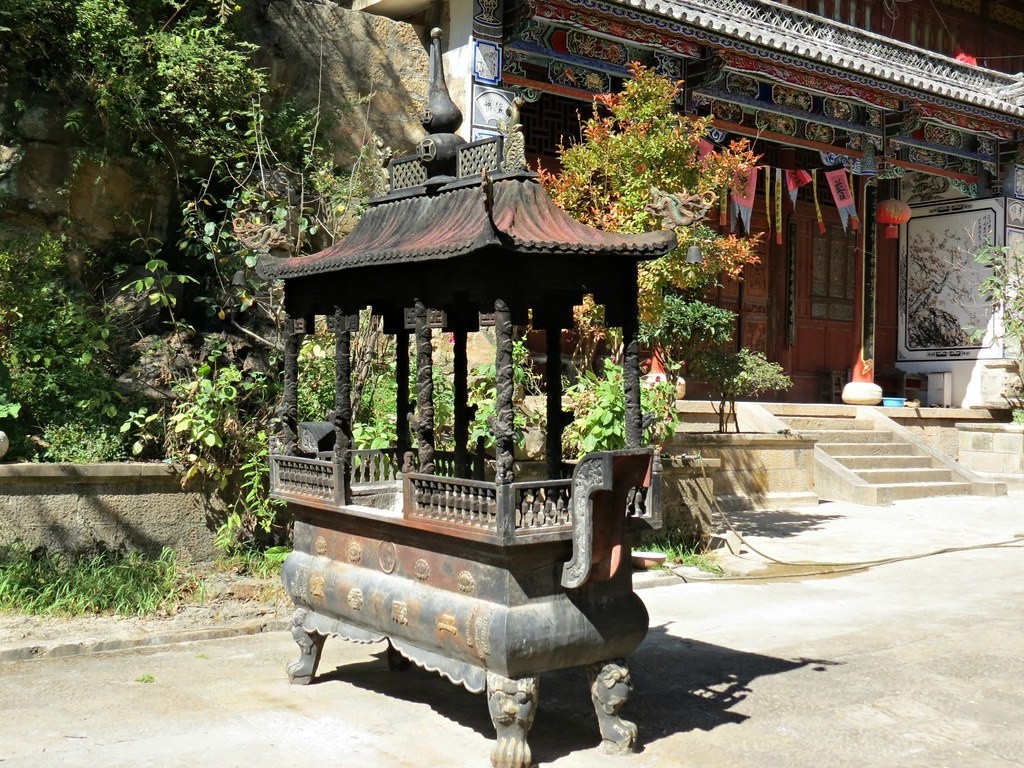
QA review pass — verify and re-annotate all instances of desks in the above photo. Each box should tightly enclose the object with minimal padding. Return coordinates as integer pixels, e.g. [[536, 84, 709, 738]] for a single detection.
[[874, 374, 928, 403]]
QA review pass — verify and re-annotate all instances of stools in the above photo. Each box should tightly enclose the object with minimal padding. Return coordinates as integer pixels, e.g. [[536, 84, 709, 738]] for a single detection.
[[816, 369, 847, 404]]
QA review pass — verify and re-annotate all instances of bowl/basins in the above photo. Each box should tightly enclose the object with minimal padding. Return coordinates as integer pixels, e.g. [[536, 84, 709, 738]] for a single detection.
[[882, 397, 907, 407]]
